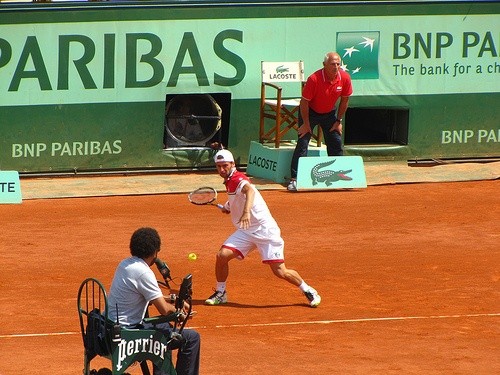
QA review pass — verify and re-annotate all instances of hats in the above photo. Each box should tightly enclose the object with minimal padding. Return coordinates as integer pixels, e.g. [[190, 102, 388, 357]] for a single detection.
[[214, 149, 234, 163]]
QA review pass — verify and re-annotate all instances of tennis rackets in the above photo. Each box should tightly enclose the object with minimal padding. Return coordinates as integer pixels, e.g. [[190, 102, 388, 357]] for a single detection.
[[188, 186, 223, 210]]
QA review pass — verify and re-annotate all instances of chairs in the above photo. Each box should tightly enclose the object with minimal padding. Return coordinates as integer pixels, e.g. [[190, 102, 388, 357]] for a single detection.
[[77, 278, 178, 375], [260, 60, 322, 148]]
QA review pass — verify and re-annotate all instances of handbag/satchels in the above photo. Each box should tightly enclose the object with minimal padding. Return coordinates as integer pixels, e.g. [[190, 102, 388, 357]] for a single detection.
[[85, 308, 115, 356]]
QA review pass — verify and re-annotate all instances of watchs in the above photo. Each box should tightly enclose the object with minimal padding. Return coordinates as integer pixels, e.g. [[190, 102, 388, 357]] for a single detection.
[[336, 118, 343, 123]]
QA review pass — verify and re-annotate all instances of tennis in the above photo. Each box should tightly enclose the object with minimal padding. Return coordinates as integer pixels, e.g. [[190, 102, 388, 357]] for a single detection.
[[188, 253, 197, 260]]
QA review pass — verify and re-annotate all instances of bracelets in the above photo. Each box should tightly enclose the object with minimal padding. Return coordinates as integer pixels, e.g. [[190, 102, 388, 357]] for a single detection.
[[224, 202, 232, 213]]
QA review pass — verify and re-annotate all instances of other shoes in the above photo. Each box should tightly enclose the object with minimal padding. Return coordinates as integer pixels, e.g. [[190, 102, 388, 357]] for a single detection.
[[288, 180, 297, 191]]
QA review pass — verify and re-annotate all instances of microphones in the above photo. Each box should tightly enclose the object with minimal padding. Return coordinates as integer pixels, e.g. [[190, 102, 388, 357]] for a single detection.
[[154, 258, 171, 280]]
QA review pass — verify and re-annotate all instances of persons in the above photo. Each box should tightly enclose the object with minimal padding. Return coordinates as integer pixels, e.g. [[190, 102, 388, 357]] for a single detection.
[[288, 52, 353, 191], [103, 227, 200, 375], [203, 149, 322, 308]]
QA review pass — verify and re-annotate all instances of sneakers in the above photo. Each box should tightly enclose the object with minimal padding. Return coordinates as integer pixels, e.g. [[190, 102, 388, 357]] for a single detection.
[[205, 287, 228, 306], [303, 286, 321, 307]]
